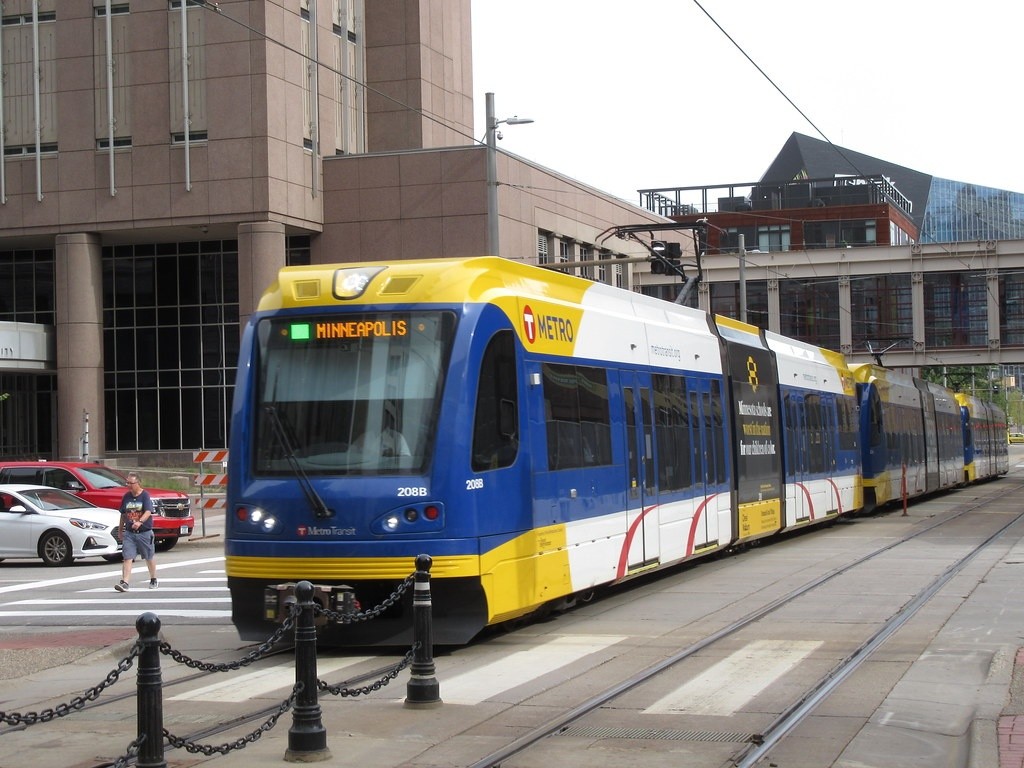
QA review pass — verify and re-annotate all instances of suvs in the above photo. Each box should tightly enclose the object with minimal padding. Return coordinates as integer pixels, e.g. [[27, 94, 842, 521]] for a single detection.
[[0, 460, 194, 553]]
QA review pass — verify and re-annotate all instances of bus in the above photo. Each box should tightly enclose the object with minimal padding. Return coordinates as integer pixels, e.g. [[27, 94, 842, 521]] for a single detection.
[[839, 336, 965, 512], [941, 369, 1010, 486], [225, 217, 866, 650]]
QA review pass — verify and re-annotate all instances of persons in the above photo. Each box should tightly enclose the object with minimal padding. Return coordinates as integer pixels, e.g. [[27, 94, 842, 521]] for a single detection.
[[114, 470, 158, 592], [347, 400, 410, 457]]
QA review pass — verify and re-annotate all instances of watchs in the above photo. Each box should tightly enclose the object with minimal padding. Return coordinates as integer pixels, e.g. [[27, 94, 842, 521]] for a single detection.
[[138, 519, 144, 524]]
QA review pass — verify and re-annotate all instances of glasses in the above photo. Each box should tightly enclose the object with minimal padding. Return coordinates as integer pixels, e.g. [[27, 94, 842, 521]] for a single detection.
[[126, 481, 138, 486]]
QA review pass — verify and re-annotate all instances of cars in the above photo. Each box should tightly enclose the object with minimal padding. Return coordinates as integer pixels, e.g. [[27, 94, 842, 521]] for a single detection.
[[0, 482, 125, 567], [1009, 433, 1024, 444]]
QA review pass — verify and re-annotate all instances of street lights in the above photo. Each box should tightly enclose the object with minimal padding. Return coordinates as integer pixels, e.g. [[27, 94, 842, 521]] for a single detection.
[[480, 91, 534, 255]]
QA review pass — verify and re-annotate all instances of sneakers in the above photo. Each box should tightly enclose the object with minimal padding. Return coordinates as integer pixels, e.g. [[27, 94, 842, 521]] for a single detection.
[[149, 579, 158, 589], [114, 580, 130, 591]]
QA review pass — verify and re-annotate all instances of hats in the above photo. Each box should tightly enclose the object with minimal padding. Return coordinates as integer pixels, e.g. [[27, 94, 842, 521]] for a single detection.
[[384, 399, 395, 420]]
